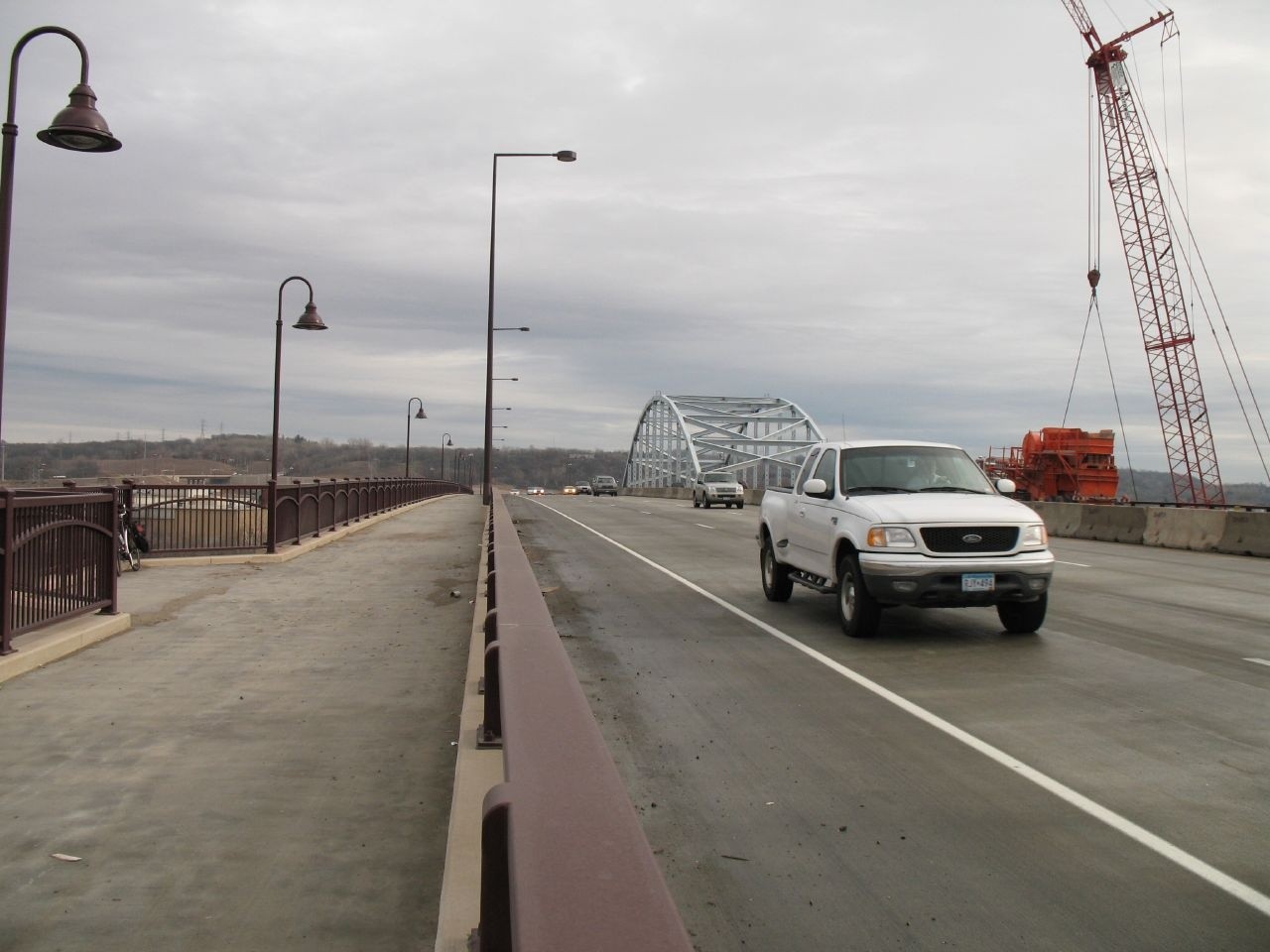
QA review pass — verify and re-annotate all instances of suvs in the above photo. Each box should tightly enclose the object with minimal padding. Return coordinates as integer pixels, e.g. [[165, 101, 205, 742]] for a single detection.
[[692, 472, 744, 509], [592, 475, 620, 497]]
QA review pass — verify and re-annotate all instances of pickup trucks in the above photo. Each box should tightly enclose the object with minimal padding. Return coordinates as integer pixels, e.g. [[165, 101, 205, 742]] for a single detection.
[[753, 441, 1055, 640]]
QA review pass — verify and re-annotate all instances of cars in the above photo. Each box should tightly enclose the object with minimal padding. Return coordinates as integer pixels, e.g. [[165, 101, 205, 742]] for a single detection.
[[509, 480, 592, 496]]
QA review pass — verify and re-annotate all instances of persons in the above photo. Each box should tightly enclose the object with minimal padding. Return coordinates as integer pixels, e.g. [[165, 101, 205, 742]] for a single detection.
[[906, 453, 951, 489]]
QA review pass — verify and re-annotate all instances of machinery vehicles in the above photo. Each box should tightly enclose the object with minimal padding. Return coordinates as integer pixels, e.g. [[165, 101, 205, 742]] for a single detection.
[[969, 1, 1269, 511]]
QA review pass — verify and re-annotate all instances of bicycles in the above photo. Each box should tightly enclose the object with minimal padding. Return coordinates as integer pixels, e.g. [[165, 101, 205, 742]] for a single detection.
[[116, 502, 142, 578]]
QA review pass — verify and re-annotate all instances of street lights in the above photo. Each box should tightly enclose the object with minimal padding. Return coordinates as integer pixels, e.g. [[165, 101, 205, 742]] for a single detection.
[[482, 150, 578, 505], [405, 397, 430, 477], [454, 449, 466, 483], [1, 22, 127, 414], [441, 433, 454, 480], [270, 270, 330, 480]]
